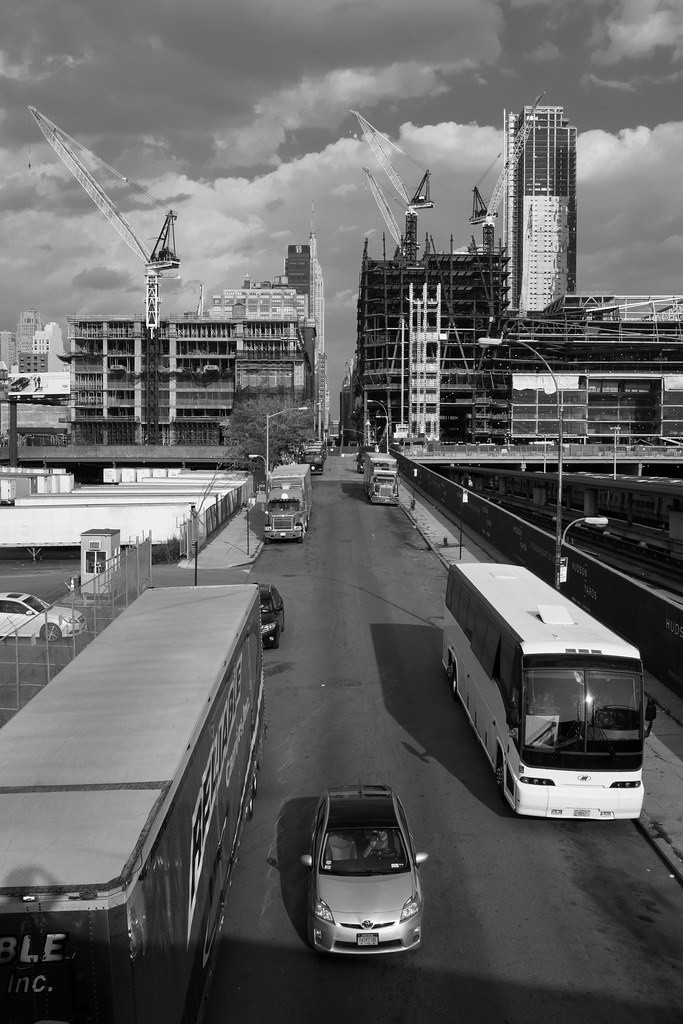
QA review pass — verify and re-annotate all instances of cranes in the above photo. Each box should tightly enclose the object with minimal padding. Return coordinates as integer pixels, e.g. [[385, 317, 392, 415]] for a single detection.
[[347, 110, 435, 244], [28, 104, 182, 273], [467, 91, 550, 227]]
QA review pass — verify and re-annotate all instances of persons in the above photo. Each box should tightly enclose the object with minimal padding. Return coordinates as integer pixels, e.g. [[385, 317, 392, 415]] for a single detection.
[[363, 829, 401, 858]]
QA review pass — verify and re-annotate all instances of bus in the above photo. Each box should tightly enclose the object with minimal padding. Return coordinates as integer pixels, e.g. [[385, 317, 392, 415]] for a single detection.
[[442, 563, 657, 820], [0, 584, 265, 1023]]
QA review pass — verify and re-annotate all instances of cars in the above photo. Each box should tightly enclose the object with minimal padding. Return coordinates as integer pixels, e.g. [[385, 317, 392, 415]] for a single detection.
[[10, 377, 30, 392], [301, 784, 429, 956], [0, 592, 87, 643], [249, 583, 284, 650], [300, 440, 327, 474]]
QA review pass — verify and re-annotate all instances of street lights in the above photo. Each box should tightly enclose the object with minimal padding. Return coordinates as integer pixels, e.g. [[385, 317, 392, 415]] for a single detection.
[[459, 472, 473, 560], [242, 501, 250, 554], [192, 539, 198, 586], [610, 426, 622, 481], [479, 335, 609, 593], [541, 434, 550, 473], [367, 399, 399, 455], [248, 407, 308, 505]]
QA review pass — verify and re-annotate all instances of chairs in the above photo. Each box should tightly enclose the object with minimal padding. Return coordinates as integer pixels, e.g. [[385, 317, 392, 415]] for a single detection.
[[326, 835, 356, 861]]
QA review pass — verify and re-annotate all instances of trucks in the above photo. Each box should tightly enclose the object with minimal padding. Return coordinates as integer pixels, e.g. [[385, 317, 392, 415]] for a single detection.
[[363, 451, 401, 505], [262, 464, 312, 545]]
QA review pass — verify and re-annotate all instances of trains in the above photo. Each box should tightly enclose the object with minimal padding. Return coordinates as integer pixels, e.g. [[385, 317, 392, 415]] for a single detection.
[[484, 470, 683, 529]]
